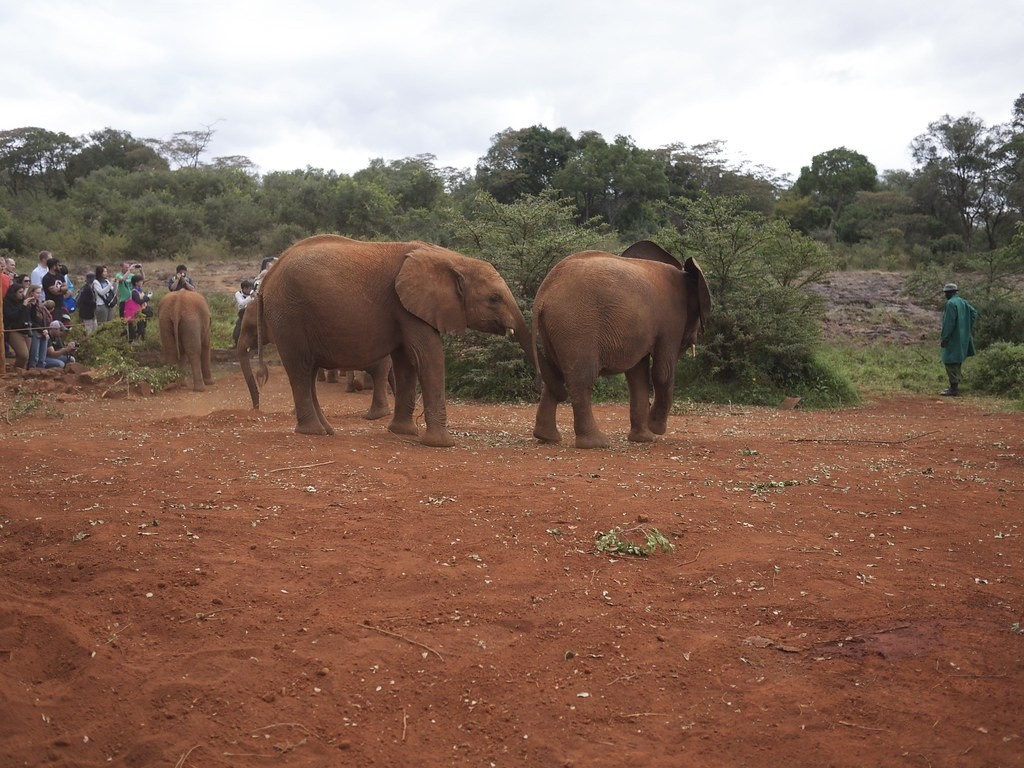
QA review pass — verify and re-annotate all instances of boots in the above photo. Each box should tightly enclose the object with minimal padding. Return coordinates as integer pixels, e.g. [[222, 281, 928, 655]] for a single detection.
[[945, 388, 950, 391], [940, 383, 959, 396]]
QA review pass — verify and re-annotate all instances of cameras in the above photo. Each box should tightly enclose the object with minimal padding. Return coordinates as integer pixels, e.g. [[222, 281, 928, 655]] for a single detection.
[[135, 265, 141, 268], [28, 297, 35, 302], [75, 341, 81, 348], [180, 273, 186, 277]]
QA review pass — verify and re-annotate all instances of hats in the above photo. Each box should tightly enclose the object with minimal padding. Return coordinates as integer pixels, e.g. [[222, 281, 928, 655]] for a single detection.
[[942, 283, 959, 293], [49, 320, 70, 331]]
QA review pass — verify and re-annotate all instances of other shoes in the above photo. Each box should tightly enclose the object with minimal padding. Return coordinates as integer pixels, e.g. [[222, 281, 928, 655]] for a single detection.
[[7, 352, 16, 358], [228, 342, 238, 348]]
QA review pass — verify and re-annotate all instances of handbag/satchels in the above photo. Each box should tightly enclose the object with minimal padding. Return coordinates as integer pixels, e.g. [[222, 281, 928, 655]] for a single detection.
[[123, 288, 143, 324], [73, 286, 84, 308], [105, 289, 118, 307], [62, 295, 77, 312]]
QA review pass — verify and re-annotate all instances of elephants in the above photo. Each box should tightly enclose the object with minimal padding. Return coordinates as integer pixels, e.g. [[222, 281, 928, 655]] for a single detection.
[[158, 288, 215, 392], [532, 240, 712, 450], [236, 233, 568, 447]]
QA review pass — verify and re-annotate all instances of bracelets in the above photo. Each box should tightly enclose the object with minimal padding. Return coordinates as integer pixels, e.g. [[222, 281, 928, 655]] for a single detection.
[[183, 282, 187, 287], [60, 286, 61, 292]]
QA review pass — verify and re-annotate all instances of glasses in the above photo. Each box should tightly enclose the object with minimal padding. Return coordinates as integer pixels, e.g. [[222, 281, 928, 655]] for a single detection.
[[24, 280, 32, 282]]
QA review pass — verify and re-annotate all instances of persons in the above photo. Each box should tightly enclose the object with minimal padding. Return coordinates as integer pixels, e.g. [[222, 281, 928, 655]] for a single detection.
[[115, 262, 145, 318], [124, 275, 153, 342], [59, 264, 75, 322], [42, 258, 68, 322], [169, 265, 196, 292], [0, 256, 19, 300], [16, 274, 31, 299], [42, 300, 55, 323], [26, 285, 51, 369], [75, 273, 98, 337], [46, 320, 77, 370], [234, 256, 280, 347], [31, 250, 52, 303], [2, 283, 37, 369], [941, 283, 978, 396], [91, 265, 119, 322]]
